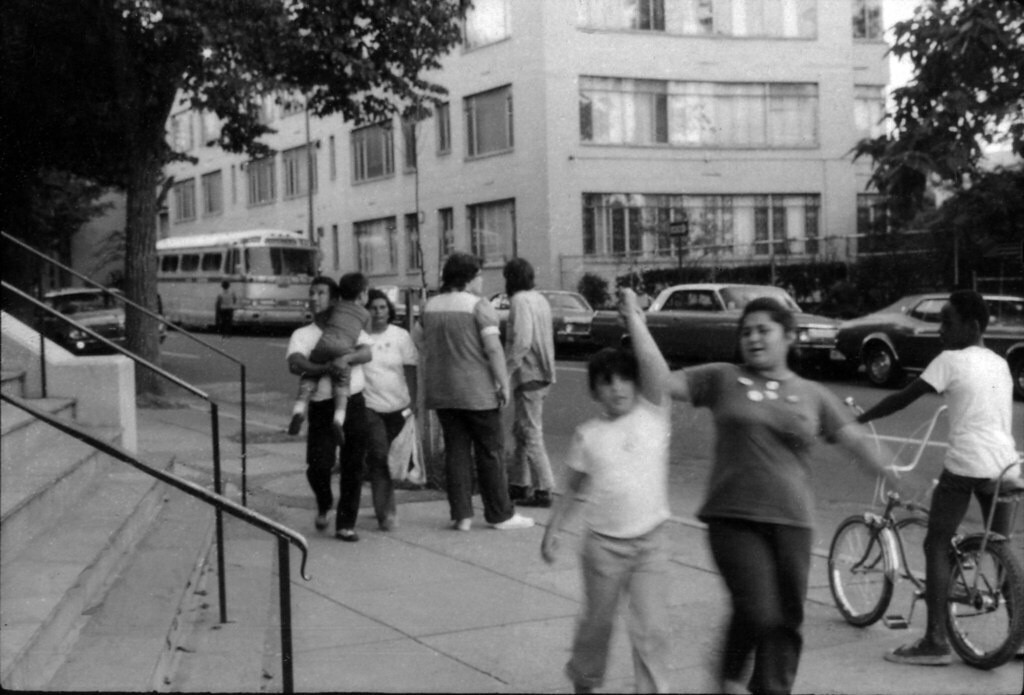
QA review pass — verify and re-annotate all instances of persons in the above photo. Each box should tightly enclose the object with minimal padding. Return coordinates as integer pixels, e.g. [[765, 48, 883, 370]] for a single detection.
[[618, 287, 926, 695], [857, 289, 1024, 665], [216, 280, 237, 342], [362, 289, 419, 529], [413, 250, 535, 531], [288, 270, 372, 446], [541, 286, 676, 694], [284, 277, 373, 541], [503, 257, 556, 507]]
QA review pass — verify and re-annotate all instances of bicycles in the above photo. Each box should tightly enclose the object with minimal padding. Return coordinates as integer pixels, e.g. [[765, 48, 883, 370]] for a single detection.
[[818, 397, 1024, 670]]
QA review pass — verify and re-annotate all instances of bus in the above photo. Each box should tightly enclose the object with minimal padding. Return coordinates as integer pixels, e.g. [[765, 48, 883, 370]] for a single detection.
[[155, 228, 326, 336]]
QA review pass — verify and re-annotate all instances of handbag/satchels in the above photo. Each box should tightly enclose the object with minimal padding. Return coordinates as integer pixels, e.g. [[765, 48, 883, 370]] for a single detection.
[[388, 414, 427, 489]]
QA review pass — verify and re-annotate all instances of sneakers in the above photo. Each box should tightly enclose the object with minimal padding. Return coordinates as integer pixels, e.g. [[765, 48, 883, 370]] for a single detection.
[[882, 637, 951, 664], [455, 518, 471, 531], [494, 513, 534, 530]]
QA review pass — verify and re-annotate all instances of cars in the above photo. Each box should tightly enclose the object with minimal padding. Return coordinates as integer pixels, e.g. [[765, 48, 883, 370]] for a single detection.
[[39, 287, 129, 356], [486, 288, 595, 352], [586, 281, 847, 376], [833, 291, 1024, 402]]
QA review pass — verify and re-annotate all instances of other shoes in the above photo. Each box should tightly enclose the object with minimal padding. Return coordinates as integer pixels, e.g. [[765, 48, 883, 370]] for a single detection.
[[336, 528, 358, 541], [332, 421, 345, 445], [507, 486, 550, 507], [315, 510, 329, 530], [379, 512, 399, 531], [288, 413, 304, 436]]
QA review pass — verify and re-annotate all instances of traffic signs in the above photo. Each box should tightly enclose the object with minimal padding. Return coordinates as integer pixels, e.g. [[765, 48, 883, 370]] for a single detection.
[[669, 220, 688, 236]]
